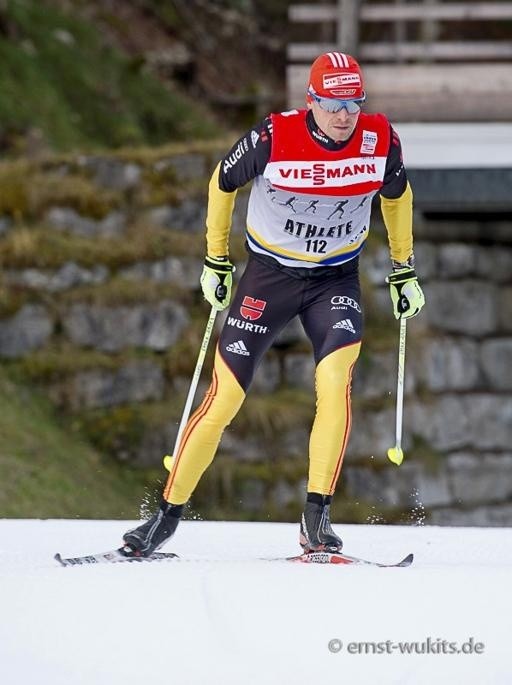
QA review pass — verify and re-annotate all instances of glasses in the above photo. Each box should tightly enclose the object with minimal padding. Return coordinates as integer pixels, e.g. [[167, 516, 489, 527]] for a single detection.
[[306, 89, 366, 116]]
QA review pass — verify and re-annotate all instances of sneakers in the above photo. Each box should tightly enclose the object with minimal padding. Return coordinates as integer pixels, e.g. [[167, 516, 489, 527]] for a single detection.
[[296, 501, 344, 554], [122, 506, 180, 560]]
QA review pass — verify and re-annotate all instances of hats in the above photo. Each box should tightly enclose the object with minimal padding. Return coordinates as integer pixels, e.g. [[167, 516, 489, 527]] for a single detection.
[[305, 50, 364, 105]]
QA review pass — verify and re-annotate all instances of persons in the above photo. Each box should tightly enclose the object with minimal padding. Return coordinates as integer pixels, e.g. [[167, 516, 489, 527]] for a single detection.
[[123, 50, 425, 554]]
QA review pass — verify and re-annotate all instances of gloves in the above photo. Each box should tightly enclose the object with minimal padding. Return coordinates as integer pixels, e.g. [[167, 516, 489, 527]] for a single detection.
[[384, 265, 427, 322], [199, 256, 235, 313]]
